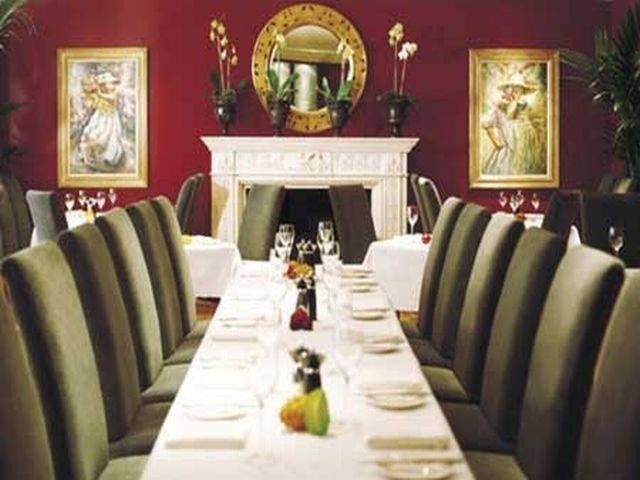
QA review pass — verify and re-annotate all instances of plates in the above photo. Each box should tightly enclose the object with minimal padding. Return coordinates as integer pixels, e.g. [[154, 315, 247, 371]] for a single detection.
[[336, 262, 459, 479], [187, 269, 270, 421]]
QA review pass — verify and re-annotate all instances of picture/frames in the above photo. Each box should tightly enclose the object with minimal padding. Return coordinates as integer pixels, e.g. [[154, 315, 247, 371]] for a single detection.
[[467, 47, 560, 188], [54, 46, 150, 189]]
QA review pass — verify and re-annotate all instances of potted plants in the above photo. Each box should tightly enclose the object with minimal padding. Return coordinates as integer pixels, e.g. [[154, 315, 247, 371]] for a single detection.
[[314, 74, 357, 137], [261, 68, 304, 136]]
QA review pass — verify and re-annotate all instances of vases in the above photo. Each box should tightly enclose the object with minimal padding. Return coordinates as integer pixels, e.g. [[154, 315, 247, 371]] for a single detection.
[[383, 97, 409, 138], [209, 92, 237, 135]]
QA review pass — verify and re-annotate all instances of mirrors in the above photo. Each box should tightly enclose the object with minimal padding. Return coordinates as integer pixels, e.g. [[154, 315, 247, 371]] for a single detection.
[[249, 1, 371, 138]]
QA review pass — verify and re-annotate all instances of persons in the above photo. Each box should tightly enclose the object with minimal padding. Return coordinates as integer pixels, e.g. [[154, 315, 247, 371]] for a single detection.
[[81, 72, 125, 171], [482, 72, 538, 176]]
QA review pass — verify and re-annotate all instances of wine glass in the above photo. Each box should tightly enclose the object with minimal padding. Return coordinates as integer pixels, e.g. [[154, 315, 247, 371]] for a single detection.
[[268, 221, 352, 322], [65, 188, 118, 215], [497, 190, 540, 219], [407, 206, 419, 236]]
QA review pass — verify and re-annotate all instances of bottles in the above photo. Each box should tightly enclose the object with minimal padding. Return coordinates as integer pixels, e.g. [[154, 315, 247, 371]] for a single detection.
[[303, 352, 323, 393]]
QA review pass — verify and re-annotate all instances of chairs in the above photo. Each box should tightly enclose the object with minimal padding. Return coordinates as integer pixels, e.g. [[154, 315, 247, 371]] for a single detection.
[[328, 181, 379, 265], [396, 164, 638, 477], [235, 182, 285, 259], [2, 163, 206, 479]]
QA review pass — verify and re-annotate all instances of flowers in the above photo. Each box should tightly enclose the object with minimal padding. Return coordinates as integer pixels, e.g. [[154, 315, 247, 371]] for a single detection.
[[202, 13, 251, 100], [375, 19, 422, 112]]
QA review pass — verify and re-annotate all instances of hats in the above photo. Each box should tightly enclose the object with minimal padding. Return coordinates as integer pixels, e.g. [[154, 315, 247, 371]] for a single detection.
[[496, 73, 536, 96]]
